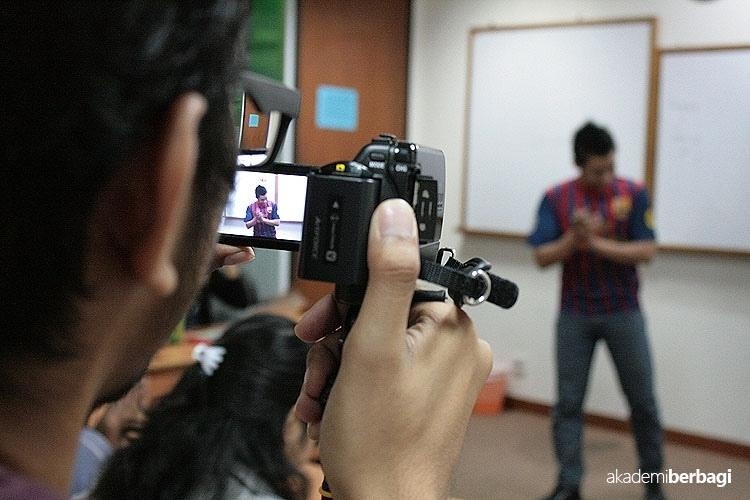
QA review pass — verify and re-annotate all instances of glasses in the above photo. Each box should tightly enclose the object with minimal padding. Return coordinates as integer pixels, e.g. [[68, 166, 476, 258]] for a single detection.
[[226, 66, 304, 173]]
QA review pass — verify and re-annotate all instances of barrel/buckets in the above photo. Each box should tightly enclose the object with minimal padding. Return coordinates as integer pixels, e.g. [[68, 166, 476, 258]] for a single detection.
[[474, 362, 508, 416]]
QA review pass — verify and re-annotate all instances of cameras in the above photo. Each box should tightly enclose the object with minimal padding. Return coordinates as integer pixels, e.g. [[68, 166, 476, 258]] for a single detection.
[[216, 133, 448, 308]]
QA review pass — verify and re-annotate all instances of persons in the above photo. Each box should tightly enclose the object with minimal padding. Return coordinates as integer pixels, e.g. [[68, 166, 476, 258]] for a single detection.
[[245, 186, 283, 240], [66, 382, 139, 499], [2, 0, 493, 500], [88, 311, 332, 499], [523, 119, 669, 500]]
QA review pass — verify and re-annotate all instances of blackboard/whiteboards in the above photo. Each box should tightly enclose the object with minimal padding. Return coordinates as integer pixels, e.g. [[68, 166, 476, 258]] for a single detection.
[[462, 16, 750, 257]]
